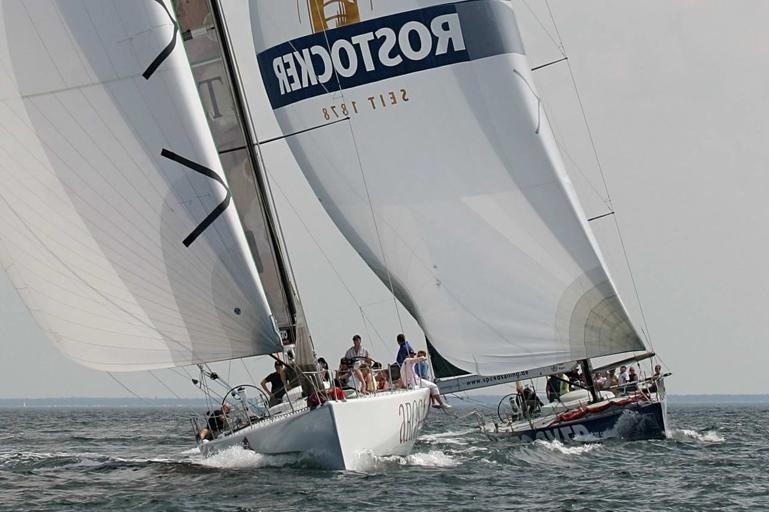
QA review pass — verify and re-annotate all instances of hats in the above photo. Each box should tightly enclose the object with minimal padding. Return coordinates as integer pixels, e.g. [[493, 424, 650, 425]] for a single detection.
[[274, 361, 282, 368]]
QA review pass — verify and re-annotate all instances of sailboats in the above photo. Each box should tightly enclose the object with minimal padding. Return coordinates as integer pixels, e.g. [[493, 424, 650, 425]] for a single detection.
[[241, 0, 677, 445], [0, 0, 442, 476]]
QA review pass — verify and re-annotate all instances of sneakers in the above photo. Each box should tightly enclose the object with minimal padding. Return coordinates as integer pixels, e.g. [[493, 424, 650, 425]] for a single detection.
[[439, 402, 448, 408], [432, 404, 440, 409]]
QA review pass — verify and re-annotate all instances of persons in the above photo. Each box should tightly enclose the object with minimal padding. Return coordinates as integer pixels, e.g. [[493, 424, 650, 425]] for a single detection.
[[197, 402, 233, 441], [260, 332, 454, 411], [512, 364, 667, 419]]
